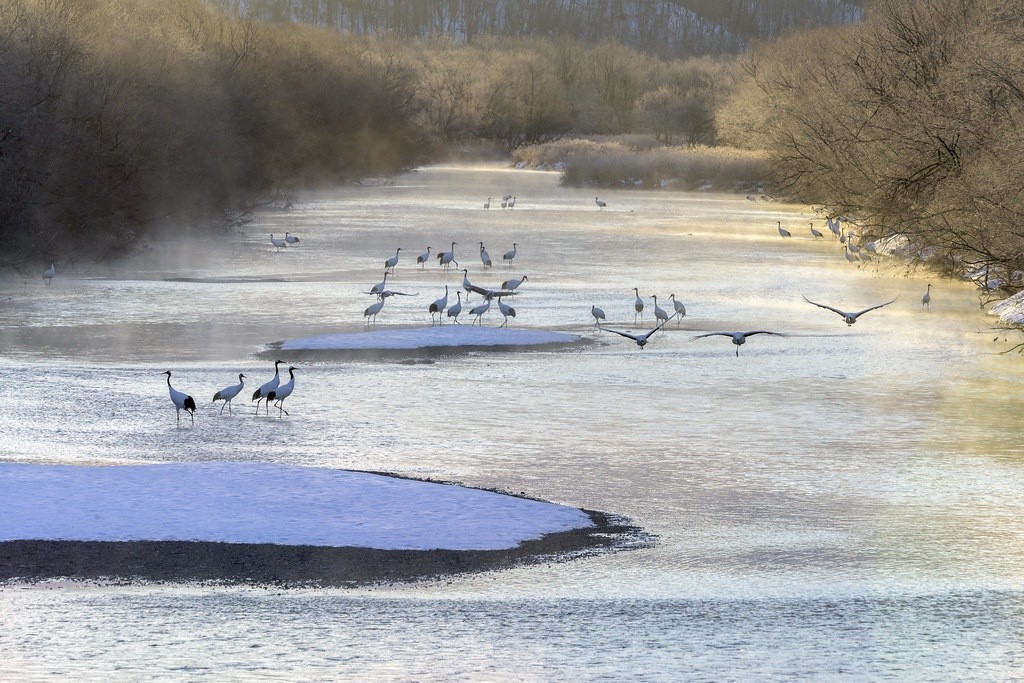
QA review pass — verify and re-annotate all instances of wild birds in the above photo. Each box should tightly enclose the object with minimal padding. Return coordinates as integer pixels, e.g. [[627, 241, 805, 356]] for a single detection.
[[802, 294, 899, 327], [213, 373, 247, 415], [269, 231, 300, 253], [159, 371, 197, 428], [592, 288, 686, 350], [807, 206, 876, 265], [360, 242, 529, 328], [594, 197, 606, 208], [688, 330, 784, 357], [500, 194, 516, 208], [483, 197, 492, 210], [776, 221, 791, 239], [252, 359, 298, 418], [42, 261, 55, 289], [921, 283, 932, 309]]
